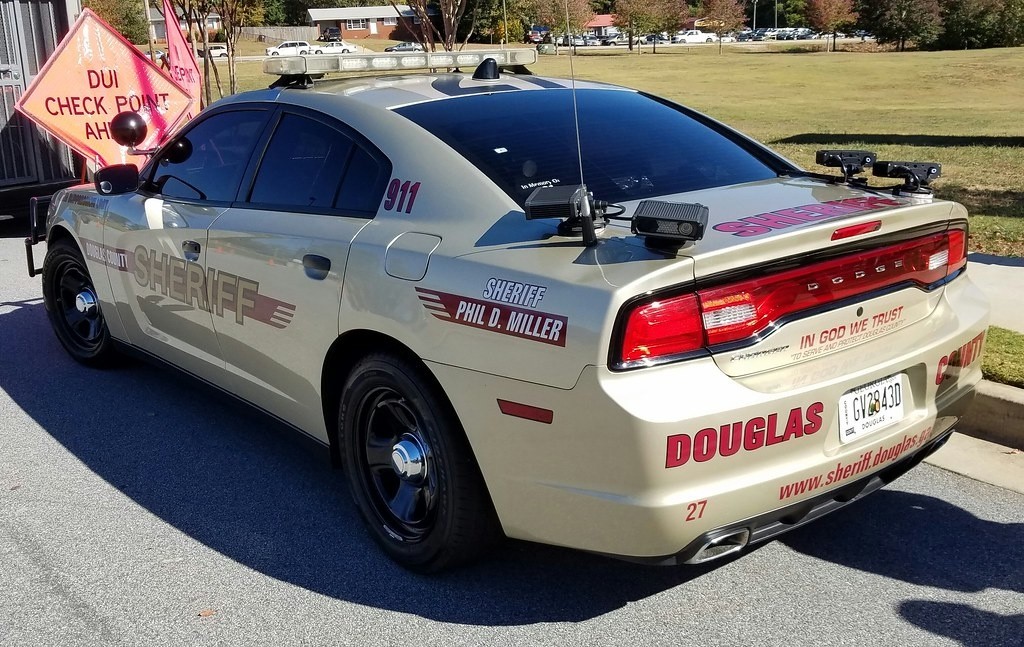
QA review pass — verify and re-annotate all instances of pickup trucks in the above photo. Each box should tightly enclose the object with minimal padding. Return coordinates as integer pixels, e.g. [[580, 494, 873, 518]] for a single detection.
[[671, 29, 717, 43]]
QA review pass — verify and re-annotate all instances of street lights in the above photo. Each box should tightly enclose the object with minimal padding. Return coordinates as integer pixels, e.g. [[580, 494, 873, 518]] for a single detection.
[[753, 0, 758, 31]]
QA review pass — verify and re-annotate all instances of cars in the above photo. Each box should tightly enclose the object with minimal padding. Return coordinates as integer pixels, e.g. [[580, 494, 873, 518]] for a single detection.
[[563, 35, 585, 46], [25, 47, 989, 577], [606, 33, 648, 46], [721, 27, 845, 42], [596, 36, 614, 46], [384, 42, 423, 52], [209, 45, 232, 57], [324, 27, 342, 42], [308, 42, 358, 54], [525, 34, 564, 46], [145, 50, 164, 60], [265, 42, 311, 56], [582, 35, 602, 46], [645, 34, 671, 44]]
